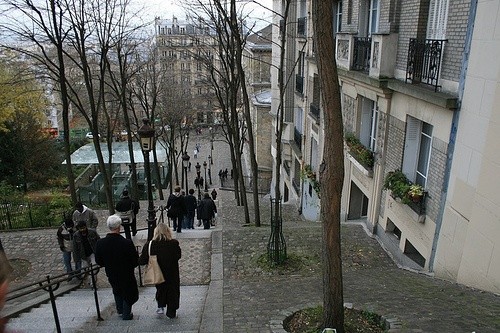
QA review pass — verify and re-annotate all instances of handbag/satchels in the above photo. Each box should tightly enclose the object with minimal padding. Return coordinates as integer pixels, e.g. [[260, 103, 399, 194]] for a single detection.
[[143, 240, 165, 287], [120, 209, 135, 225]]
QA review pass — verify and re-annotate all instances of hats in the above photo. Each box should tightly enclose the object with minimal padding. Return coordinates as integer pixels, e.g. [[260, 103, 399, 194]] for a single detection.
[[123, 190, 129, 196]]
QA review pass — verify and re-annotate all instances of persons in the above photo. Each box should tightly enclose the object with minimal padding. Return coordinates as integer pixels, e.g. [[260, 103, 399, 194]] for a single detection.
[[56, 218, 83, 281], [139, 223, 181, 318], [73, 220, 101, 288], [198, 192, 217, 228], [72, 202, 99, 231], [167, 186, 184, 232], [187, 140, 228, 192], [211, 188, 217, 199], [115, 190, 139, 239], [95, 214, 139, 320], [184, 188, 197, 229]]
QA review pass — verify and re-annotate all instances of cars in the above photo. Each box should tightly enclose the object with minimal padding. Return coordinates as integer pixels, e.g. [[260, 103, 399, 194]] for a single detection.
[[85, 131, 100, 138]]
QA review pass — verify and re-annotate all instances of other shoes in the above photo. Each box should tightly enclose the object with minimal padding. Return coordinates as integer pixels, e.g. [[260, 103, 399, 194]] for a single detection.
[[122, 312, 133, 320], [155, 308, 165, 313]]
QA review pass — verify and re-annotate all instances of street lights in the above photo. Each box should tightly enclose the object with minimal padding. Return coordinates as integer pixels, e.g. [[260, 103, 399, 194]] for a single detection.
[[181, 152, 190, 195], [195, 162, 201, 202], [203, 161, 208, 192], [138, 118, 156, 237], [207, 155, 212, 185]]
